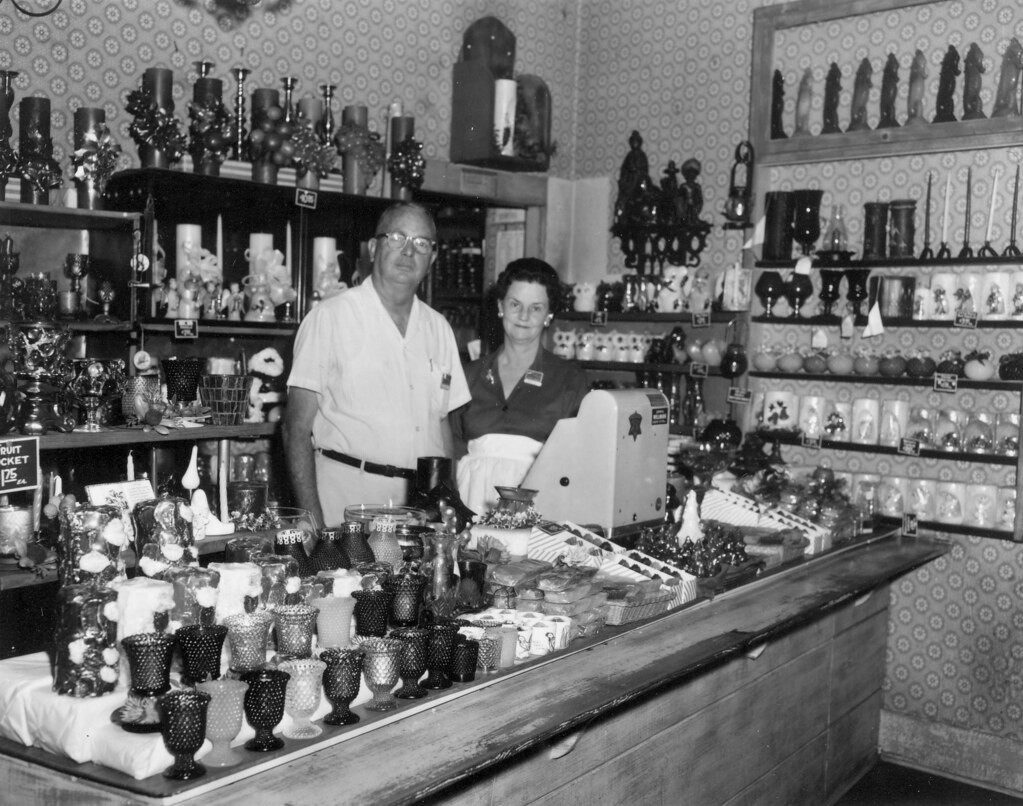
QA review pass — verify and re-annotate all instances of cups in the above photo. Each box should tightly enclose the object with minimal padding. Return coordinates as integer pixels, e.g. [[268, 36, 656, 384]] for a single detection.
[[58, 290, 81, 320], [930, 271, 1023, 320], [160, 355, 207, 401], [869, 275, 914, 320], [229, 480, 268, 520], [198, 373, 253, 424], [752, 391, 1021, 532]]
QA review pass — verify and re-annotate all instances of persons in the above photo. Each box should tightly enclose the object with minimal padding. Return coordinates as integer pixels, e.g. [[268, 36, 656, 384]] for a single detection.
[[452, 258, 588, 459], [283, 203, 471, 530]]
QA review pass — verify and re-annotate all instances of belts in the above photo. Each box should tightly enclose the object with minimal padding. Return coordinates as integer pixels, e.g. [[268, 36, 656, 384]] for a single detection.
[[317, 449, 416, 480]]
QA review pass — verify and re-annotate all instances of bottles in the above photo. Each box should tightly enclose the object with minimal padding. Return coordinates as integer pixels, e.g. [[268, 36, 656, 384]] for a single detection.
[[275, 517, 404, 578], [822, 203, 848, 251]]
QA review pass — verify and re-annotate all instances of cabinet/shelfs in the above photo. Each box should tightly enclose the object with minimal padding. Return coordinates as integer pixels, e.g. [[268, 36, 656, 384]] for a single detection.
[[747, 259, 1023, 545], [554, 311, 745, 436], [83, 167, 404, 495], [0, 201, 281, 591]]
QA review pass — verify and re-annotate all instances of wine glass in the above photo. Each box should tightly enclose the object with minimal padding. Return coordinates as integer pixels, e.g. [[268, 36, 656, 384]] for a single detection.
[[793, 189, 824, 267], [755, 268, 871, 320], [118, 554, 572, 782]]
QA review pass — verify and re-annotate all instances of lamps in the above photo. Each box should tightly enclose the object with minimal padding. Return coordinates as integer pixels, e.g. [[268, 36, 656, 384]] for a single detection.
[[721, 141, 754, 231]]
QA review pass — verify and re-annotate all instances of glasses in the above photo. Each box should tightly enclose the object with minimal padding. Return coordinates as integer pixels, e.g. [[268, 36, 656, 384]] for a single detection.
[[375, 232, 437, 255]]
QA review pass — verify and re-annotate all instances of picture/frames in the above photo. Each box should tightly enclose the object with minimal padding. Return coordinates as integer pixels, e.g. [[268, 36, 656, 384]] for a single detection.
[[748, 0, 1023, 169]]
